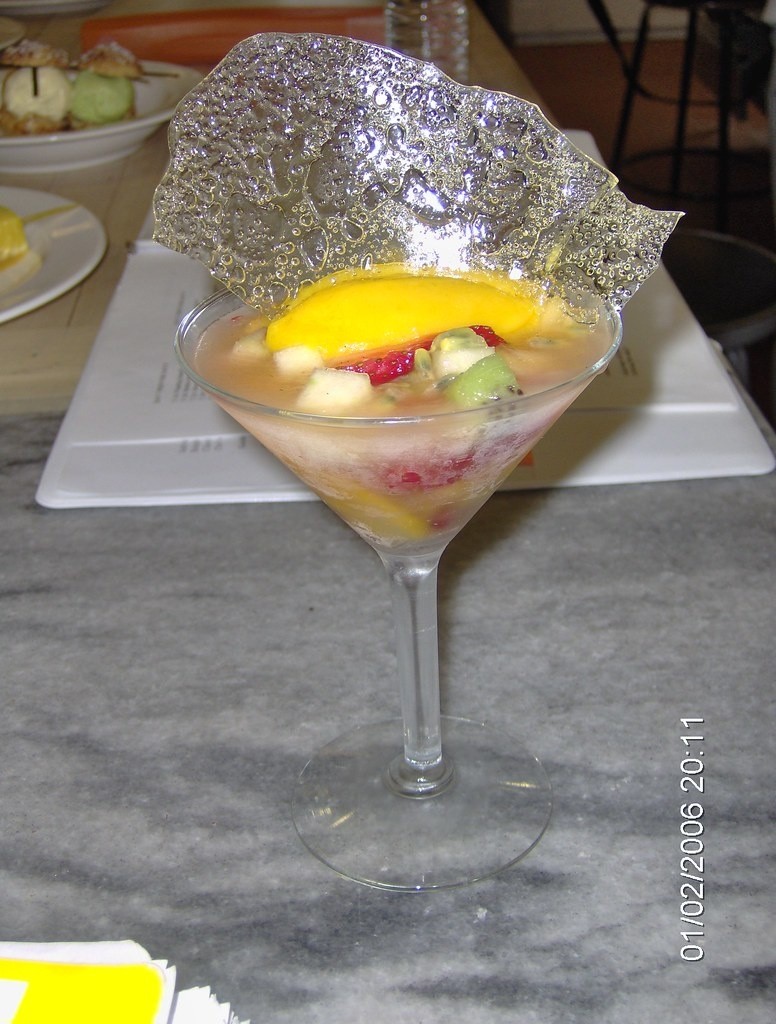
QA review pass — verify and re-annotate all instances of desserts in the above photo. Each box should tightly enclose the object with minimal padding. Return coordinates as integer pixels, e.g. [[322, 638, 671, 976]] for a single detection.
[[0, 204, 29, 266], [0, 36, 147, 135]]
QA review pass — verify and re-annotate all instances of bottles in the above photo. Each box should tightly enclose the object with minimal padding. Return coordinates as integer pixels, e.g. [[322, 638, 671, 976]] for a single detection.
[[383, 0, 471, 86]]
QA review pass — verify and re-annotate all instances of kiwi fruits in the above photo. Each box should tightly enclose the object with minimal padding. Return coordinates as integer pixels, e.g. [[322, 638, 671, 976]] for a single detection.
[[442, 352, 526, 411]]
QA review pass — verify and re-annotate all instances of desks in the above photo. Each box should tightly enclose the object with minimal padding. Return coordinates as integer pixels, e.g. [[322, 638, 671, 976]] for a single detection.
[[1, 0, 775, 1024]]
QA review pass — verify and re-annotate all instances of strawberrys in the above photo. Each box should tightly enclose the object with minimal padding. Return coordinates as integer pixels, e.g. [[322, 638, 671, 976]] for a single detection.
[[335, 324, 509, 385]]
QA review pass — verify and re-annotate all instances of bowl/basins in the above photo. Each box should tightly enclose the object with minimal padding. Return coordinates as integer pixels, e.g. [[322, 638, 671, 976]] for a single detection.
[[0, 58, 202, 176]]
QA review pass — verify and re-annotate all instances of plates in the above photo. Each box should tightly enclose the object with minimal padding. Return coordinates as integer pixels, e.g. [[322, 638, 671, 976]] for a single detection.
[[0, 185, 107, 323]]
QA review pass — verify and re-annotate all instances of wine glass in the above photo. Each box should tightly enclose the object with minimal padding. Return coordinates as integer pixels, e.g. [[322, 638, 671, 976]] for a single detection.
[[170, 273, 623, 891]]
